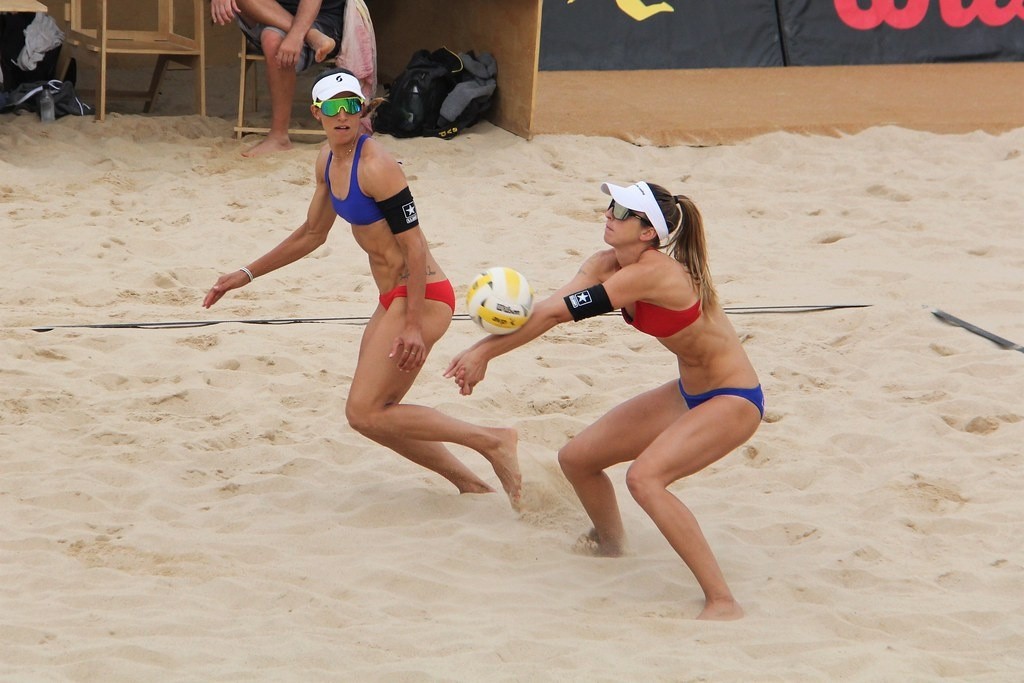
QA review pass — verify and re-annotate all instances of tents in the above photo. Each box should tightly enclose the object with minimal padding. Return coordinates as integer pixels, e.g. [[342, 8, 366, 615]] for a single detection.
[[371, 0, 1024, 146]]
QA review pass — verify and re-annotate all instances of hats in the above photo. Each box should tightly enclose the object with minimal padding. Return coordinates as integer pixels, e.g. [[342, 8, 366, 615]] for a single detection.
[[600, 181, 668, 241], [311, 73, 366, 102]]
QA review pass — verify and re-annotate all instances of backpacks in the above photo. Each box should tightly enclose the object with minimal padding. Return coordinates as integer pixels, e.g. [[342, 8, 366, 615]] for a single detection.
[[378, 47, 459, 141]]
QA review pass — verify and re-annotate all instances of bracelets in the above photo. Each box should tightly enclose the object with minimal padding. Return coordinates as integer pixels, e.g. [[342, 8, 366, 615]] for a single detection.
[[240, 268, 253, 282]]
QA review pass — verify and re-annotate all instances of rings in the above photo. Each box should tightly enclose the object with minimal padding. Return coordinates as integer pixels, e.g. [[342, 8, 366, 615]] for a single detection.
[[411, 352, 416, 355], [404, 349, 410, 353]]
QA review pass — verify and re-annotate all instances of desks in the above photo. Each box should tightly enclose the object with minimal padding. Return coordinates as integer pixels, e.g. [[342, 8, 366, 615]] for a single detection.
[[0, 0, 49, 13]]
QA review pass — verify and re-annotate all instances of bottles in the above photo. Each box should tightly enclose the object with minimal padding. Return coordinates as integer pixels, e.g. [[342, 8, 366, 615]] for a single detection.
[[40, 90, 55, 124]]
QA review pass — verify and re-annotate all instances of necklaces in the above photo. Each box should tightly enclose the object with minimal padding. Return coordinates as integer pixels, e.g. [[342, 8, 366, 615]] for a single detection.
[[332, 136, 355, 159]]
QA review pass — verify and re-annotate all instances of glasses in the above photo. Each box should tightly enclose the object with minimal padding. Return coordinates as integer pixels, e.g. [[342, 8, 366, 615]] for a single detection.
[[313, 97, 364, 117], [607, 199, 649, 225]]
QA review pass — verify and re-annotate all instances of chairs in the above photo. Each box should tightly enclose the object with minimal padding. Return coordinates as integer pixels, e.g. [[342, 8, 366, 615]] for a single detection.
[[232, 35, 338, 148], [53, 0, 212, 126]]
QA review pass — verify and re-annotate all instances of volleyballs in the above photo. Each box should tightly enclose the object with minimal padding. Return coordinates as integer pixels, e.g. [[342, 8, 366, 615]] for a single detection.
[[465, 267, 533, 336]]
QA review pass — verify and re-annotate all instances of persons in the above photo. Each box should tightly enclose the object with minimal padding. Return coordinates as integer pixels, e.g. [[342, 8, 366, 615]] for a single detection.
[[211, 0, 346, 157], [203, 70, 522, 513], [444, 179, 765, 622]]
